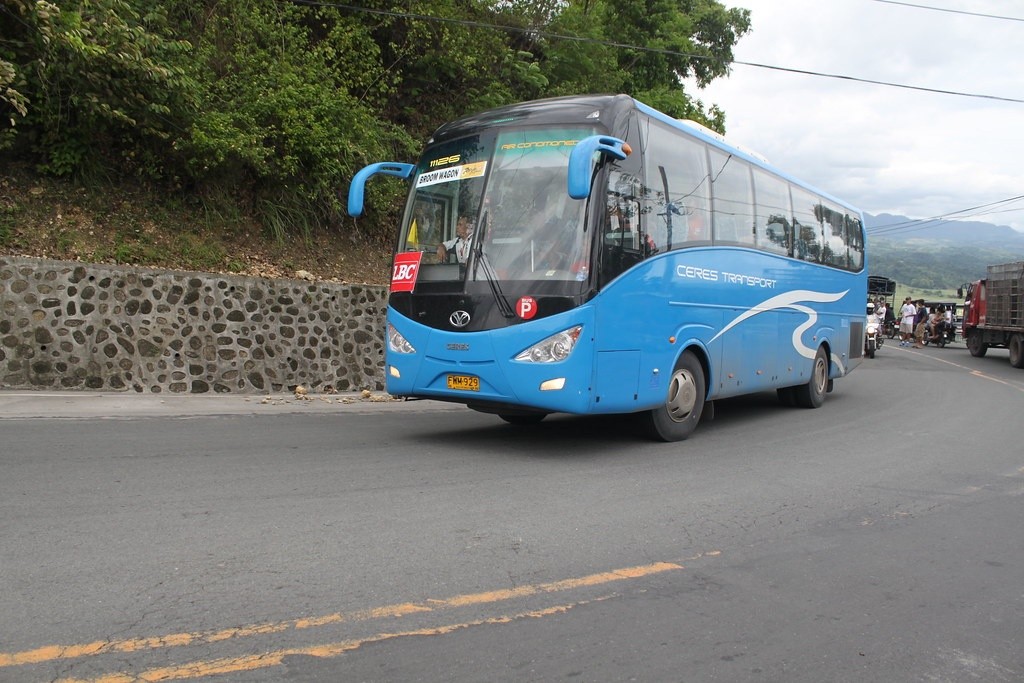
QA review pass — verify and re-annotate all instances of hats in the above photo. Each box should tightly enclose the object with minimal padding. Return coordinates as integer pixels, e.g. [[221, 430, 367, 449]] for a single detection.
[[905, 296, 912, 300], [867, 302, 875, 308]]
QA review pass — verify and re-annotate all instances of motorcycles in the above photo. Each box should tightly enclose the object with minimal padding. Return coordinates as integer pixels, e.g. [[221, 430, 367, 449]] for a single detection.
[[866, 322, 884, 359], [921, 328, 948, 348]]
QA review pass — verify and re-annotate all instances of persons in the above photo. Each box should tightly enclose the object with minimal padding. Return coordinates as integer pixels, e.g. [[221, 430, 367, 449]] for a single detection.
[[930, 303, 952, 338], [866, 302, 881, 333], [884, 303, 894, 327], [438, 212, 484, 281], [913, 299, 928, 349], [539, 236, 570, 272], [952, 303, 958, 321], [867, 296, 884, 314], [928, 308, 936, 320], [876, 300, 886, 321], [898, 297, 917, 347]]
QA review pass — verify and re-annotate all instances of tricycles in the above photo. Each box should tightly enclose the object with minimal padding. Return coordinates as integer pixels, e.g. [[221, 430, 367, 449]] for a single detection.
[[881, 320, 904, 341]]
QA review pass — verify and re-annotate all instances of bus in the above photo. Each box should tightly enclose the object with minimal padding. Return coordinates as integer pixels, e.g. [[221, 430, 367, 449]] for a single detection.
[[348, 94, 867, 442]]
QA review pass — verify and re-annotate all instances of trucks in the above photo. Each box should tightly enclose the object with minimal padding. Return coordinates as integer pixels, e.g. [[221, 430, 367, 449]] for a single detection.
[[957, 261, 1024, 368]]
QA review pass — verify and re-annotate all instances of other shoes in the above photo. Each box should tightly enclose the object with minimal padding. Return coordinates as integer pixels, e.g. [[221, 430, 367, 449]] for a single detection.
[[906, 343, 909, 347], [898, 343, 906, 347], [916, 346, 922, 349]]
